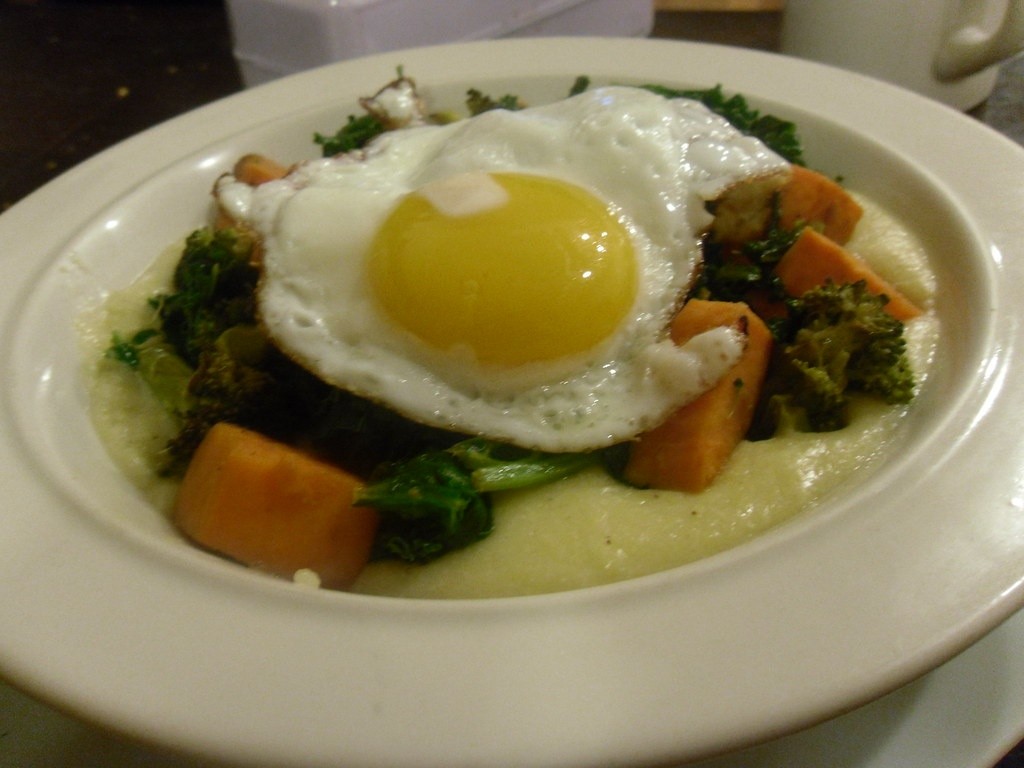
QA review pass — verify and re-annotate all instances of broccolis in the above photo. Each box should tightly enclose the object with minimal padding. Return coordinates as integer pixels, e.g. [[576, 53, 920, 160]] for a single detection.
[[770, 277, 917, 430]]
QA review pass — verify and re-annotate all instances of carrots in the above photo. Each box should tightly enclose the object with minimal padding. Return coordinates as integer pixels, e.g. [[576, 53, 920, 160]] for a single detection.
[[761, 165, 862, 247], [623, 297, 775, 490], [742, 230, 924, 330], [175, 419, 381, 588]]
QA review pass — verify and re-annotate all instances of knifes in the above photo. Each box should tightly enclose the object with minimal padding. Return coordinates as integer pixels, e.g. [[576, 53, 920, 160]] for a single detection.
[[932, 20, 1023, 85]]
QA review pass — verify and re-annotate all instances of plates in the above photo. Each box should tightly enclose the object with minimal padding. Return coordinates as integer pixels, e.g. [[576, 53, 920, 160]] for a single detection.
[[0, 35, 1024, 768]]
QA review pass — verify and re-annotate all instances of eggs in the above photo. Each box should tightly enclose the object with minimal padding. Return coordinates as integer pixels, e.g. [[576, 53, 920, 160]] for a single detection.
[[210, 86, 790, 455]]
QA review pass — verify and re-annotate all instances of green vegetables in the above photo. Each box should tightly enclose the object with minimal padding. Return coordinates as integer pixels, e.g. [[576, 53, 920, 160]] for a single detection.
[[107, 79, 813, 562]]
